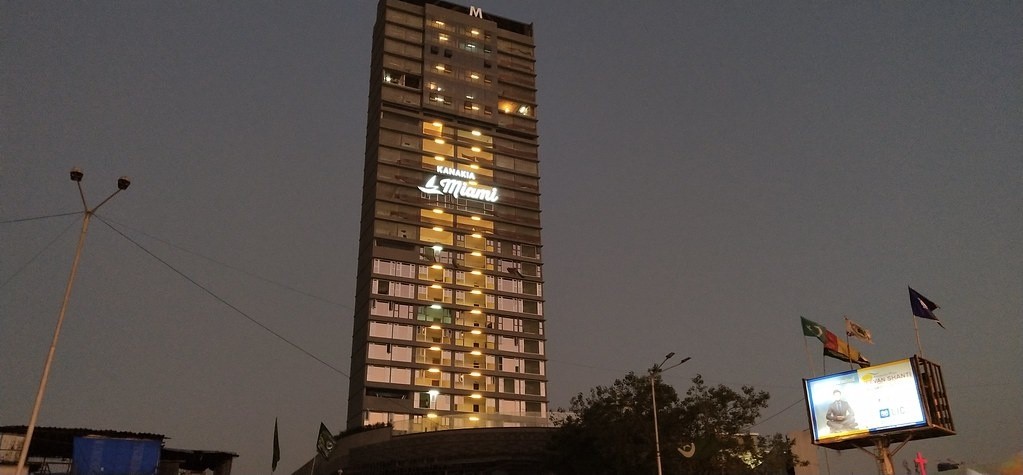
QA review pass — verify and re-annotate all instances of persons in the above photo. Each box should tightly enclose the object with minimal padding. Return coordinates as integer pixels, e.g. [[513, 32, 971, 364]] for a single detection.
[[826, 390, 858, 433]]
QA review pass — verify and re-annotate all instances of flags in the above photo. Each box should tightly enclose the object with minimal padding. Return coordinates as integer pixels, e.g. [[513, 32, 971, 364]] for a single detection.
[[271, 420, 280, 472], [909, 286, 939, 322], [823, 330, 858, 363], [845, 319, 871, 343], [315, 422, 338, 461], [801, 316, 826, 344]]
